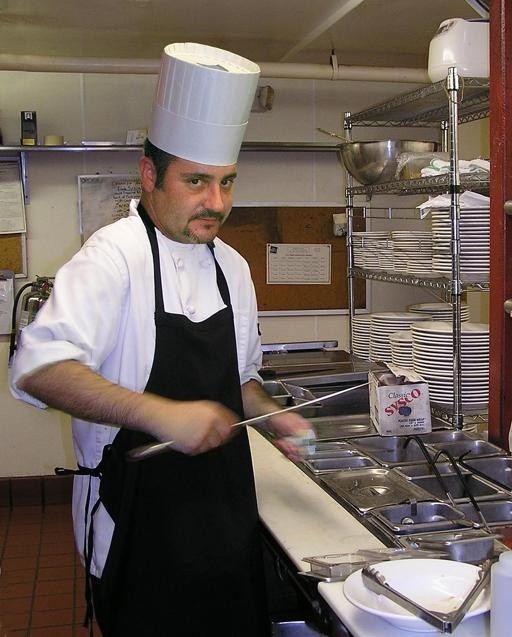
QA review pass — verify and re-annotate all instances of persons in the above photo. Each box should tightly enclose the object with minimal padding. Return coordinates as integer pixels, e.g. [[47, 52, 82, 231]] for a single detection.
[[6, 138, 315, 637]]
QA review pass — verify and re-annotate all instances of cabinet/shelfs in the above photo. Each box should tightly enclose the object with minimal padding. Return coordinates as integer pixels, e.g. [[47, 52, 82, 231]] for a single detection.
[[341, 66, 490, 431]]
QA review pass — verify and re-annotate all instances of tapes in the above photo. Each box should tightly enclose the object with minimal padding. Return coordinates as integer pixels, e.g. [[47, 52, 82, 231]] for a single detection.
[[44, 135, 64, 146]]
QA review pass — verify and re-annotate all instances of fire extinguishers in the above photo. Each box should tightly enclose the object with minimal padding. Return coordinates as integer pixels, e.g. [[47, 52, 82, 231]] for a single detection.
[[8, 275, 55, 368]]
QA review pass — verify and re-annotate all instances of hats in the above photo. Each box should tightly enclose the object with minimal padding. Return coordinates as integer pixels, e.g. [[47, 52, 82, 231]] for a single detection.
[[145, 41, 262, 167]]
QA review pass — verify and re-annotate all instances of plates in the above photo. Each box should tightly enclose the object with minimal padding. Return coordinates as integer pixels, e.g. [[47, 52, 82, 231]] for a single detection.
[[351, 302, 490, 411], [353, 205, 490, 283], [343, 558, 491, 630]]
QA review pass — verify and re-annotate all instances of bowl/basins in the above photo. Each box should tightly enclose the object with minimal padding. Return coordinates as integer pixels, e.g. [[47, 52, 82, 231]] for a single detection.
[[335, 139, 441, 185]]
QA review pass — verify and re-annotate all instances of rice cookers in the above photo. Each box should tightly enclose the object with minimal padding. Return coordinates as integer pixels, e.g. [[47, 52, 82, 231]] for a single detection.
[[426, 1, 491, 81]]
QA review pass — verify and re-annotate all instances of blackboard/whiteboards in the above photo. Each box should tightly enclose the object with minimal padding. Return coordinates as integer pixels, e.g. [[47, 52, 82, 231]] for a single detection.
[[78, 173, 143, 248]]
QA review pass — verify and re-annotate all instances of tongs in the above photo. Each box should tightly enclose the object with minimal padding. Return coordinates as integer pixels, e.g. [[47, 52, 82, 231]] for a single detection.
[[360, 558, 495, 632]]
